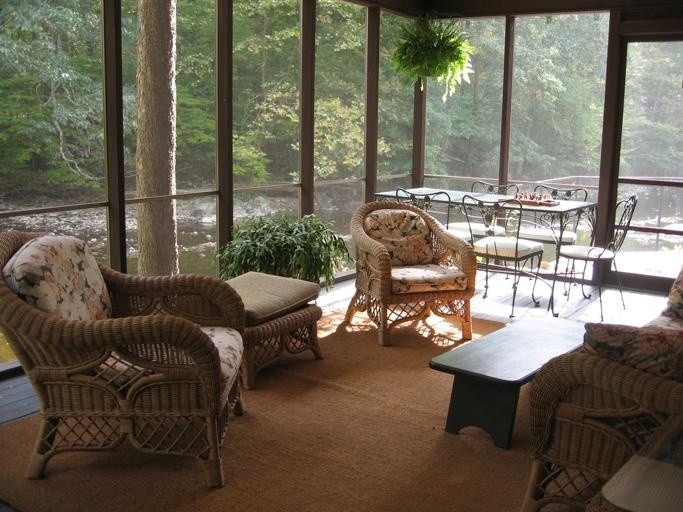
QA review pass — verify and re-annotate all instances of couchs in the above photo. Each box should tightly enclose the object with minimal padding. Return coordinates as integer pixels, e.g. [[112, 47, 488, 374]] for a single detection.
[[519, 270, 683, 512]]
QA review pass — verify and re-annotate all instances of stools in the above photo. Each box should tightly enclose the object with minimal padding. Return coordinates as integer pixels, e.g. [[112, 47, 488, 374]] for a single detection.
[[224, 271, 327, 390]]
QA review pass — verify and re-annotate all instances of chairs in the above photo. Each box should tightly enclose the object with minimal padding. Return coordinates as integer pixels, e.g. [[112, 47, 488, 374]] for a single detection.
[[369, 182, 638, 321], [347, 201, 478, 348], [0, 226, 248, 487]]
